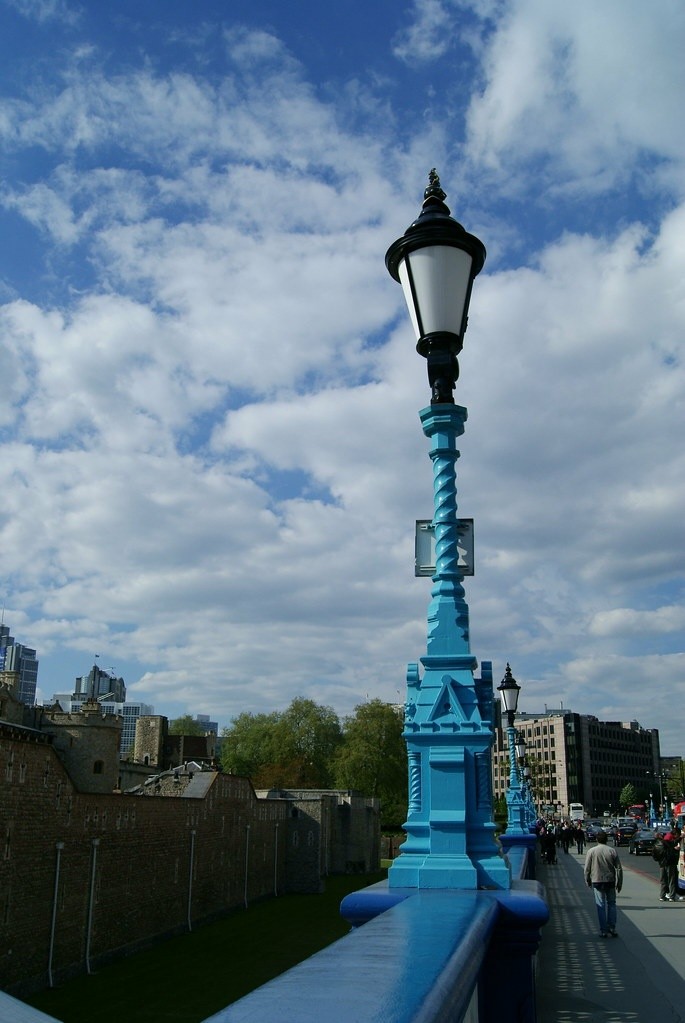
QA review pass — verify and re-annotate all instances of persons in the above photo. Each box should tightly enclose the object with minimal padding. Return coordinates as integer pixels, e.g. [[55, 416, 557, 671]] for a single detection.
[[555, 819, 582, 854], [670, 819, 678, 829], [540, 820, 557, 865], [584, 832, 622, 937], [537, 818, 542, 832], [574, 825, 586, 855], [656, 828, 681, 901], [613, 831, 619, 846]]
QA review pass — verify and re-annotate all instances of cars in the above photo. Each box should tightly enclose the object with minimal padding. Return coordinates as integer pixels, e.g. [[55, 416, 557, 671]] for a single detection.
[[585, 810, 672, 843], [627, 830, 657, 856], [612, 827, 636, 847]]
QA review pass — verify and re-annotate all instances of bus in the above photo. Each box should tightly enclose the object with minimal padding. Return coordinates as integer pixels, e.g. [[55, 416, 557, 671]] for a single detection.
[[669, 801, 685, 890], [624, 804, 646, 822], [569, 803, 584, 823]]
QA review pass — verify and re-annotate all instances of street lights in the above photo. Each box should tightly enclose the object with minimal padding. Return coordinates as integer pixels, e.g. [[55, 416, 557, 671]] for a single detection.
[[649, 791, 656, 820], [381, 164, 515, 890], [513, 732, 537, 834], [663, 796, 670, 821], [496, 661, 531, 836]]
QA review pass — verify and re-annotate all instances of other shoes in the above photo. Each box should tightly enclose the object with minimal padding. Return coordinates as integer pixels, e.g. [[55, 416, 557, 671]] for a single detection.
[[608, 927, 618, 937], [669, 898, 678, 902], [659, 898, 669, 901], [599, 932, 607, 937]]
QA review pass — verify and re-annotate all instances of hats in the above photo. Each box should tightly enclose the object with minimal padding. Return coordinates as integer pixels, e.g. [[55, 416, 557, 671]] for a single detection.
[[664, 833, 677, 841]]
